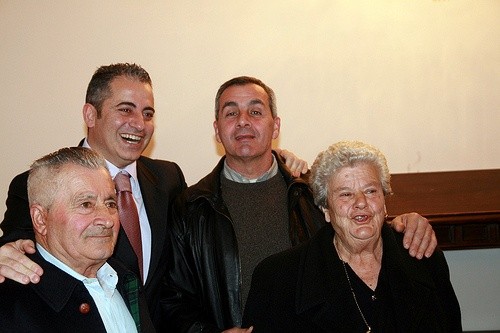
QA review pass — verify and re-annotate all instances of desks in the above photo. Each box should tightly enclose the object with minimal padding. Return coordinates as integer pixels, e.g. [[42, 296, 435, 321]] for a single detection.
[[386, 168, 499, 250]]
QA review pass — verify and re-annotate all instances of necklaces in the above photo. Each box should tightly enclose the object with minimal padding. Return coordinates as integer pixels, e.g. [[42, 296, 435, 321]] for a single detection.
[[334, 233, 377, 333]]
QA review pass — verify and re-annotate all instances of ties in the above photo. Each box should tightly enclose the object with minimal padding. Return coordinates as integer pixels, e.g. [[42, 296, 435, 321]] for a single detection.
[[112, 173, 144, 285]]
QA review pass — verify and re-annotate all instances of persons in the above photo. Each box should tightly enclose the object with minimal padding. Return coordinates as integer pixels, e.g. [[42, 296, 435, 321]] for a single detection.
[[242, 139, 463, 333], [0, 62, 189, 333], [139, 76, 438, 333], [0, 147, 156, 333]]
